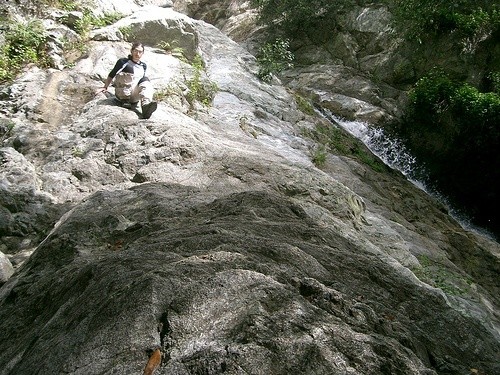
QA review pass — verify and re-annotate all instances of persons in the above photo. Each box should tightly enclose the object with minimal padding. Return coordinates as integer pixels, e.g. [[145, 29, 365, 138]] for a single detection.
[[96, 42, 157, 119]]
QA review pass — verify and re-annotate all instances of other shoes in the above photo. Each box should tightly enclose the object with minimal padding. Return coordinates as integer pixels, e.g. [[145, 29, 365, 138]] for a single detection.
[[142, 100, 158, 119]]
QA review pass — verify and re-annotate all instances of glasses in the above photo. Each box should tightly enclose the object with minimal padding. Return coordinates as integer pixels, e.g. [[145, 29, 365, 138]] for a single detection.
[[132, 49, 144, 54]]
